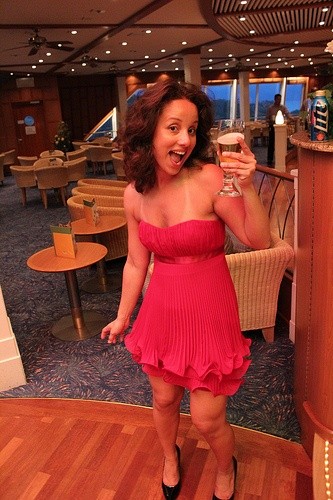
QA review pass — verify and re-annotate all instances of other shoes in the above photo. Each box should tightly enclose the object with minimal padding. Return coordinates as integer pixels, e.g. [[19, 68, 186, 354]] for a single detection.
[[266, 159, 272, 164]]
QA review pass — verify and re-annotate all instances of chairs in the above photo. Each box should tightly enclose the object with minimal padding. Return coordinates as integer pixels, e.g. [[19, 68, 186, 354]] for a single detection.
[[66, 178, 128, 263], [207, 119, 268, 157], [0, 136, 126, 210], [142, 230, 294, 342]]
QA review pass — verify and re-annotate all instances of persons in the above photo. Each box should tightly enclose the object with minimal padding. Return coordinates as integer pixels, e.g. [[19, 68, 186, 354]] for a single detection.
[[102, 80, 269, 500], [266, 93, 293, 167]]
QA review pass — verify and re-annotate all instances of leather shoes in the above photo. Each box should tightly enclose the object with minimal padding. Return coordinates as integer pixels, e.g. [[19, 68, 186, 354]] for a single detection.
[[161, 446, 181, 500], [212, 455, 237, 500]]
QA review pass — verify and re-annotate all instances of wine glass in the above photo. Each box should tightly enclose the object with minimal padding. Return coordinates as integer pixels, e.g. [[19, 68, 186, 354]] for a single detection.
[[215, 119, 246, 197]]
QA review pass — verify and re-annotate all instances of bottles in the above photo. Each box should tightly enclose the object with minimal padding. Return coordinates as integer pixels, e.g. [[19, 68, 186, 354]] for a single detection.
[[310, 90, 330, 142]]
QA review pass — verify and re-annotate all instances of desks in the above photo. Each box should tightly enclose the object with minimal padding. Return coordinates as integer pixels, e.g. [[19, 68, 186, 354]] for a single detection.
[[65, 215, 128, 294], [26, 242, 108, 342]]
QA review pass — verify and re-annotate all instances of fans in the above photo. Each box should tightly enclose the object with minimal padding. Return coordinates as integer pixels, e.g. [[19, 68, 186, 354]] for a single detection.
[[1, 25, 74, 57]]
